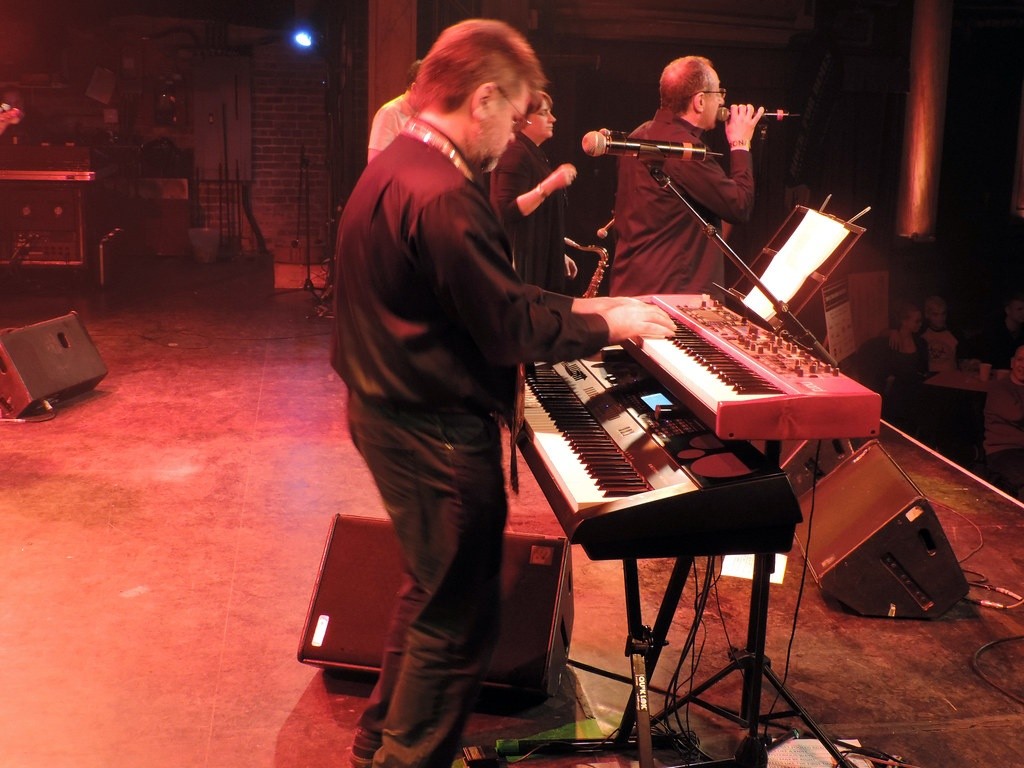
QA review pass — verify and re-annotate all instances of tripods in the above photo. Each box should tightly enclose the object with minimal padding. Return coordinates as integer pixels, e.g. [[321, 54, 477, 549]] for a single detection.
[[267, 146, 328, 306], [647, 442, 852, 768]]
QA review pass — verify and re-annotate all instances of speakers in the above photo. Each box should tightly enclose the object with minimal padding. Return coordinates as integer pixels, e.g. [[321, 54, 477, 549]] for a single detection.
[[750, 436, 969, 619], [298, 512, 576, 702], [0, 311, 109, 420]]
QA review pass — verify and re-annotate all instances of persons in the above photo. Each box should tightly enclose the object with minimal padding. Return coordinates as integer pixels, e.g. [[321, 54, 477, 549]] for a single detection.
[[369, 58, 423, 165], [610, 54, 765, 299], [869, 293, 1024, 507], [490, 91, 577, 294], [332, 20, 679, 768]]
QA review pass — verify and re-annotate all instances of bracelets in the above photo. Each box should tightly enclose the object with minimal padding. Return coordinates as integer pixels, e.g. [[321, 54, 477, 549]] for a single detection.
[[730, 139, 751, 149]]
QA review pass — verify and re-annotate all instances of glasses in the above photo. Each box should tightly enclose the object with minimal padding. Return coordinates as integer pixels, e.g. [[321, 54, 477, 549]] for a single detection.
[[499, 87, 532, 131], [688, 89, 726, 99]]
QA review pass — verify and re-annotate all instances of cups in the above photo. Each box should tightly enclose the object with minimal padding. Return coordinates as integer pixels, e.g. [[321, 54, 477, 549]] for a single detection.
[[980, 362, 992, 383], [996, 370, 1009, 380]]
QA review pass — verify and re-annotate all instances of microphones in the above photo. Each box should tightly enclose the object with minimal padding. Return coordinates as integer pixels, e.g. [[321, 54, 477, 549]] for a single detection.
[[581, 128, 725, 163], [716, 106, 800, 122], [597, 219, 614, 239]]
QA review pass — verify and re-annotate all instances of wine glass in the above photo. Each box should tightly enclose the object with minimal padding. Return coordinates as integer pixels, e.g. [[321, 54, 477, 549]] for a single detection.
[[958, 358, 981, 382]]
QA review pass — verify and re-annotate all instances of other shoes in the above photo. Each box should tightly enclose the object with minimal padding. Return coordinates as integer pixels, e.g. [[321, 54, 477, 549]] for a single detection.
[[350, 728, 382, 768]]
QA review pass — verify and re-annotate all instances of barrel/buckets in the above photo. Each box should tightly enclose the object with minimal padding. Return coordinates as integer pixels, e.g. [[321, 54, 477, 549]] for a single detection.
[[186, 228, 221, 266]]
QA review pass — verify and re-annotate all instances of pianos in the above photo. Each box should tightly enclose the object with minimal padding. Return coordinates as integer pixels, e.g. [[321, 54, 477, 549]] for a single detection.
[[519, 290, 882, 767]]
